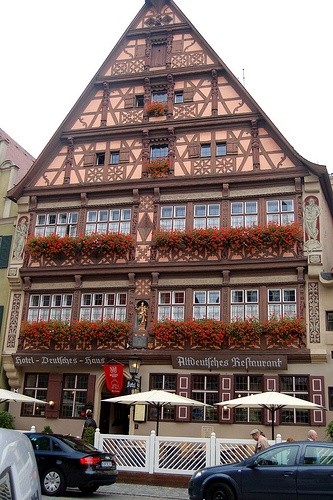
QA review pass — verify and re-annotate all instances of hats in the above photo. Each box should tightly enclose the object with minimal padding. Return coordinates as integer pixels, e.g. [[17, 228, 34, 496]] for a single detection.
[[250, 429, 258, 435]]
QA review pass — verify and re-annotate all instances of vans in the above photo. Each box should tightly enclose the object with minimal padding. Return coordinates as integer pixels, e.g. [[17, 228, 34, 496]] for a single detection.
[[0, 426, 42, 500]]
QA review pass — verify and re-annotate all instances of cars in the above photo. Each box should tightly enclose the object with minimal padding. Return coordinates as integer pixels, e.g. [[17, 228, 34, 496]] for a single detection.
[[21, 433, 119, 498], [188, 442, 333, 500]]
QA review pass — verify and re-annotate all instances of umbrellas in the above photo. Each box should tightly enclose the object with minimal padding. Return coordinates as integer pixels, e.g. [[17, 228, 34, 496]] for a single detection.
[[0, 388, 48, 412], [214, 391, 323, 440], [100, 390, 217, 436]]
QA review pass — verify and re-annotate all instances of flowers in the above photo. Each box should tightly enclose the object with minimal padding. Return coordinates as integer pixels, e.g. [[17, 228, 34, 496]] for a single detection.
[[149, 309, 309, 347], [144, 155, 171, 178], [149, 221, 307, 253], [25, 231, 137, 258], [19, 318, 133, 347], [143, 100, 168, 116]]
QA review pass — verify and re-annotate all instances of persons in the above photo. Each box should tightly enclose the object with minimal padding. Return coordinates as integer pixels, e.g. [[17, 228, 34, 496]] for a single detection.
[[250, 429, 269, 454], [306, 430, 318, 441], [81, 409, 97, 439]]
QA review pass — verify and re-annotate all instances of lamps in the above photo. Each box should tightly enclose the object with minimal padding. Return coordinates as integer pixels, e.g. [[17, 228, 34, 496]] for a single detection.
[[127, 356, 142, 393]]
